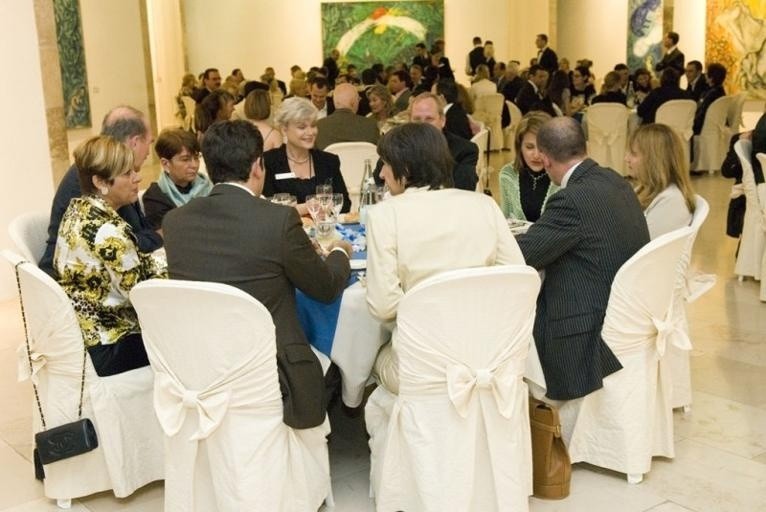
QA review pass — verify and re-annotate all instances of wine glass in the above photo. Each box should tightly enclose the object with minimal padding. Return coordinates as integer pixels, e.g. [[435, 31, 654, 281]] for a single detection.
[[272, 183, 345, 250]]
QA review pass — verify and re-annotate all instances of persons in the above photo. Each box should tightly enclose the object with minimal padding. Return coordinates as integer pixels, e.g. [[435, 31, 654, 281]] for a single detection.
[[158, 118, 352, 430], [47, 134, 165, 377], [263, 97, 352, 214], [510, 115, 651, 400], [500, 113, 560, 225], [364, 120, 525, 395], [373, 91, 479, 191], [141, 126, 214, 230], [35, 104, 165, 278], [625, 123, 697, 242], [142, 28, 728, 163], [750, 112, 766, 184]]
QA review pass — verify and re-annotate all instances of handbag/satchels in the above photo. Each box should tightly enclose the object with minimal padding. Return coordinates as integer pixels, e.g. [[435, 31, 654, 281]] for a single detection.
[[35, 417, 98, 466], [527, 396, 571, 500]]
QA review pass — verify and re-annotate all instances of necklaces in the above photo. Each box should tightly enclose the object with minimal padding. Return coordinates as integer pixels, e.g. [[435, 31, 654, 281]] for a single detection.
[[527, 170, 548, 190]]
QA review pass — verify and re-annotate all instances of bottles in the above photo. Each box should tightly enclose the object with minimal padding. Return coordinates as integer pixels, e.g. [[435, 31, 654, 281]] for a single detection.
[[359, 159, 377, 209], [626, 81, 636, 109]]
